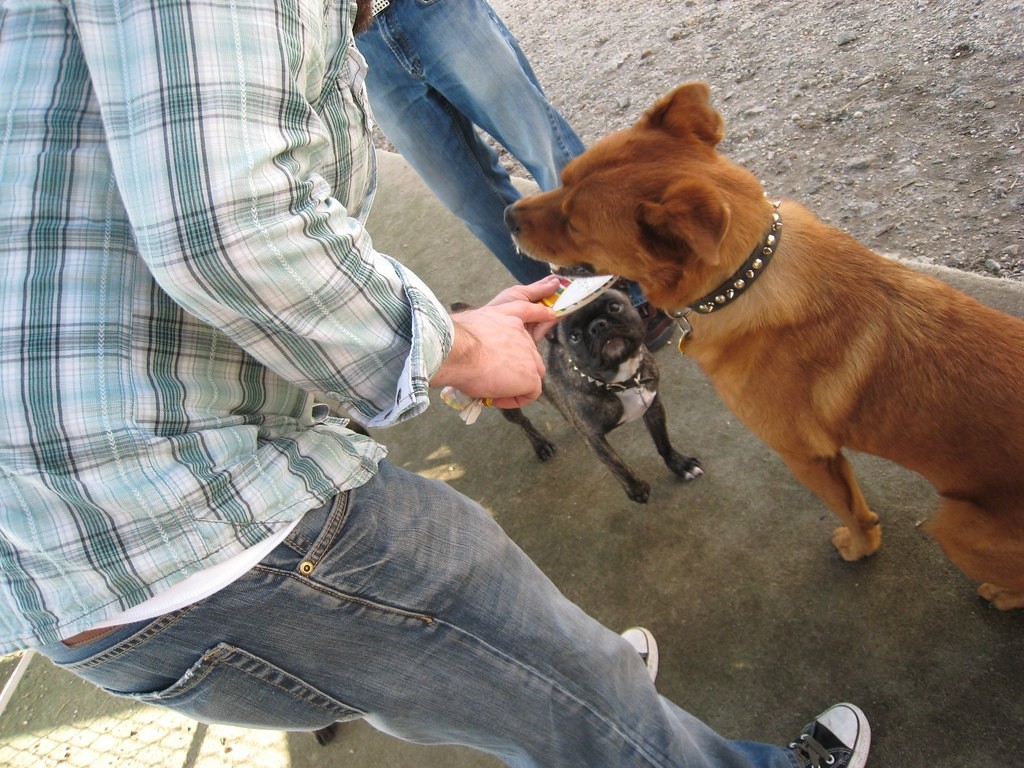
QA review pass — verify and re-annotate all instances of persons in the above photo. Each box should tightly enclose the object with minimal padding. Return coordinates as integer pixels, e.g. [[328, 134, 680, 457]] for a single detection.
[[0, 0, 872, 768], [353, 0, 679, 354]]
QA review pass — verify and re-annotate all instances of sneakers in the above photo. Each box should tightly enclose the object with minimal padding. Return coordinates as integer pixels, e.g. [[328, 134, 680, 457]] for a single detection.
[[787, 703, 872, 768], [620, 626, 658, 683]]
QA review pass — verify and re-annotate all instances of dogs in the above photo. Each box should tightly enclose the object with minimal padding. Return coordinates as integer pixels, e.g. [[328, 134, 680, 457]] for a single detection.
[[450, 82, 1024, 612]]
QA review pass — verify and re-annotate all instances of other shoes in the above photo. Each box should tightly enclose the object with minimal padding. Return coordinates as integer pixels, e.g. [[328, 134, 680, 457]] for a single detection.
[[636, 300, 676, 352]]
